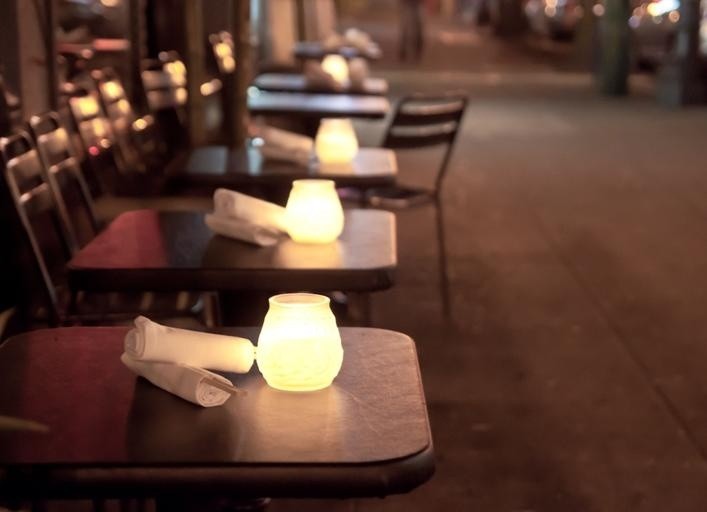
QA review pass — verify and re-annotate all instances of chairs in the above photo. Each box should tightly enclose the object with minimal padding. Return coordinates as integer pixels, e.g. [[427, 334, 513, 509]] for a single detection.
[[2, 28, 399, 332], [335, 93, 469, 326]]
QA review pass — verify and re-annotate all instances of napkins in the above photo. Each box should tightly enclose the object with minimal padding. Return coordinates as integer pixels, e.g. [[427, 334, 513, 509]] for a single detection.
[[124, 317, 256, 375], [116, 335, 237, 407]]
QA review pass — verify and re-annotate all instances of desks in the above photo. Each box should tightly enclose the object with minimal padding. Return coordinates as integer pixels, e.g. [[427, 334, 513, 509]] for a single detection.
[[3, 326, 436, 509]]
